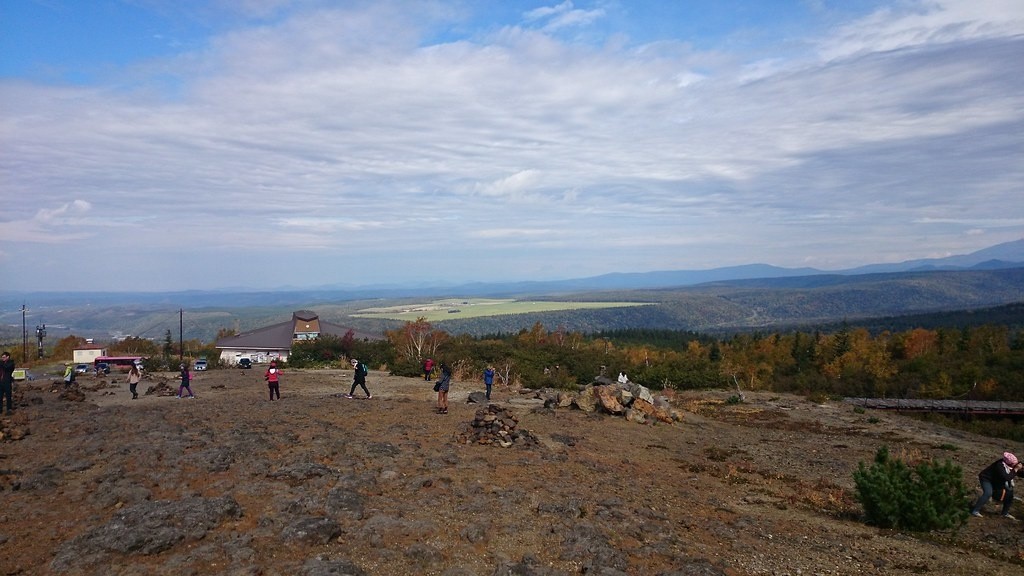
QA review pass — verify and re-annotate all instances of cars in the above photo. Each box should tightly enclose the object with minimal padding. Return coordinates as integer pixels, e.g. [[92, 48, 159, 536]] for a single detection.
[[239, 358, 252, 369], [94, 363, 110, 374], [75, 364, 89, 373], [194, 360, 207, 371]]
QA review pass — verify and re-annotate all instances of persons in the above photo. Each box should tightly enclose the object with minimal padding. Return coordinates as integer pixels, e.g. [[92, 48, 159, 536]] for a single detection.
[[264, 362, 283, 401], [0, 352, 15, 416], [175, 363, 194, 400], [345, 359, 372, 400], [972, 452, 1024, 519], [618, 371, 629, 384], [126, 363, 141, 400], [435, 361, 450, 414], [484, 365, 495, 401], [421, 357, 436, 381], [63, 362, 72, 389]]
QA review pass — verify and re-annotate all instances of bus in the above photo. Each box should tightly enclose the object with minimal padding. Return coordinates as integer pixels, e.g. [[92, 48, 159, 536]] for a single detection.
[[94, 356, 145, 372]]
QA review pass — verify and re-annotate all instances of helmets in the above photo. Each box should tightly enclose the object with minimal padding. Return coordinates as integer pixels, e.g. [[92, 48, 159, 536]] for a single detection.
[[64, 362, 71, 366]]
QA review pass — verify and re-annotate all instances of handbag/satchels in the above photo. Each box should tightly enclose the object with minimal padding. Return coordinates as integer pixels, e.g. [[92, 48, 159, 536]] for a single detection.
[[433, 381, 443, 392]]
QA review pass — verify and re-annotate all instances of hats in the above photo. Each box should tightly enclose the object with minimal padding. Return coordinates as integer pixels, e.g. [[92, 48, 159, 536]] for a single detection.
[[1002, 451, 1019, 466], [271, 363, 276, 366]]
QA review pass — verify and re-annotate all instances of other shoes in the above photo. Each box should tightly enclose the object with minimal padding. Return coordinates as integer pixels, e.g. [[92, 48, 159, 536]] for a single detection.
[[276, 397, 280, 401], [175, 395, 182, 400], [436, 410, 444, 414], [188, 395, 195, 399], [443, 409, 448, 415], [972, 511, 983, 518], [344, 394, 353, 399], [269, 398, 275, 402], [366, 395, 373, 400], [132, 396, 138, 400], [1002, 513, 1017, 521]]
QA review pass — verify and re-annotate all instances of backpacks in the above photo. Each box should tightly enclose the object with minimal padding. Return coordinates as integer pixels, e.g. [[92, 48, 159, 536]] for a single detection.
[[361, 364, 369, 377]]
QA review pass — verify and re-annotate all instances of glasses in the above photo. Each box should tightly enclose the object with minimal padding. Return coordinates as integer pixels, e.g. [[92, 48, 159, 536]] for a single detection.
[[352, 361, 358, 364]]
